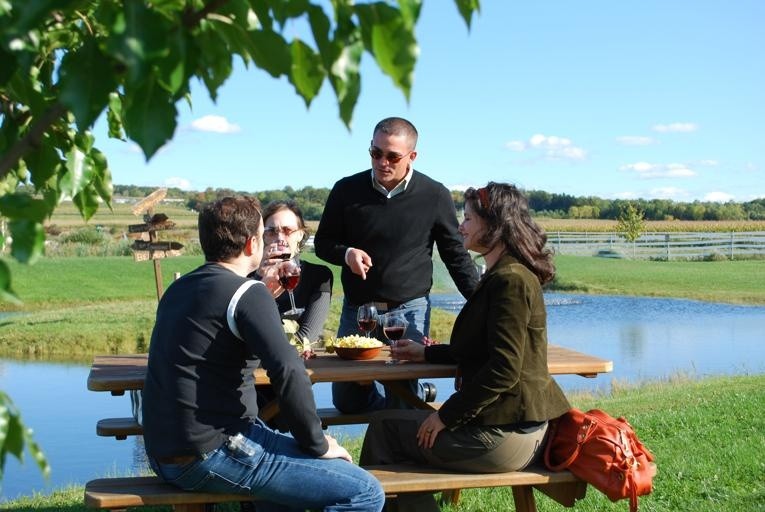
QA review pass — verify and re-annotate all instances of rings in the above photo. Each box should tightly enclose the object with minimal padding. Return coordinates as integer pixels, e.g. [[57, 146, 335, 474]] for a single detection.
[[426, 429, 431, 434]]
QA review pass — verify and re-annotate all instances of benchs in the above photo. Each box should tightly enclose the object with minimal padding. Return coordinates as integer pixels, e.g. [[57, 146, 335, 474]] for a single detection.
[[97, 401, 448, 441], [84, 466, 579, 512]]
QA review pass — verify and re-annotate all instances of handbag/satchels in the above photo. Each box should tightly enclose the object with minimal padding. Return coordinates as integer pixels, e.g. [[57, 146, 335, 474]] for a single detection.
[[543, 408, 656, 501]]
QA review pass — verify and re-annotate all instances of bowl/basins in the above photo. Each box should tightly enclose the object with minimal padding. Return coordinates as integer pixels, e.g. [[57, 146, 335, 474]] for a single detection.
[[332, 344, 386, 361]]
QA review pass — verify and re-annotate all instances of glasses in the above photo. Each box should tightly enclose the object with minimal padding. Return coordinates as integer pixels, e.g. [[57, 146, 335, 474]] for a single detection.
[[264, 225, 301, 238], [368, 300, 401, 312], [368, 143, 413, 163]]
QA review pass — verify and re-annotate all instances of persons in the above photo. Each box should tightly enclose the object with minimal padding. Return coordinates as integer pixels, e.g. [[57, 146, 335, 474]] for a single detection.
[[361, 181, 573, 511], [247, 197, 333, 422], [314, 116, 481, 414], [141, 192, 387, 511]]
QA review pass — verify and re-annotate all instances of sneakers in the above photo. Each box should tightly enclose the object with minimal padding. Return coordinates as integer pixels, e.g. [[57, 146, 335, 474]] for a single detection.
[[421, 382, 436, 402]]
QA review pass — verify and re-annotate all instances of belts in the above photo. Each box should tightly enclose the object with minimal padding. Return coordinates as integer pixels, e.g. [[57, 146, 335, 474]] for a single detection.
[[154, 453, 176, 466]]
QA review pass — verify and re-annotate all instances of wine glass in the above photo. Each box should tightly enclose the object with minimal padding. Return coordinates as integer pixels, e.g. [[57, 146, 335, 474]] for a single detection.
[[381, 312, 407, 367], [270, 239, 291, 262], [356, 304, 379, 339], [273, 257, 306, 317]]
